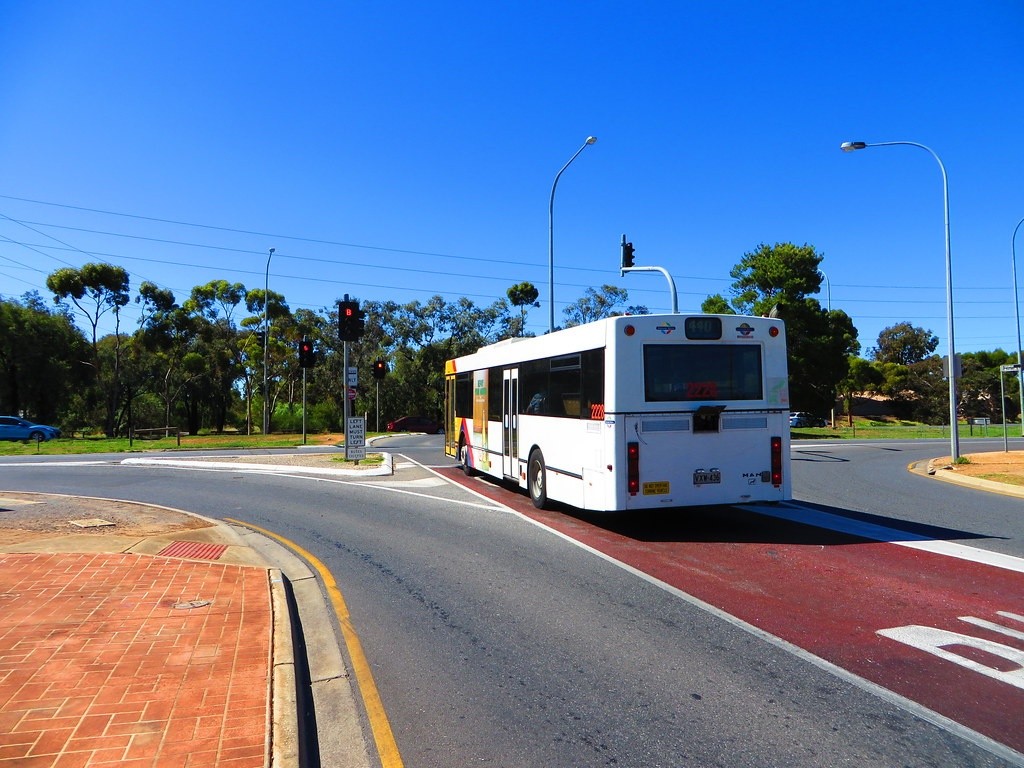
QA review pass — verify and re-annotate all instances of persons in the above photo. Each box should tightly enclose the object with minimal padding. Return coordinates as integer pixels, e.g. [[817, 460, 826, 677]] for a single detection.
[[526, 385, 548, 414]]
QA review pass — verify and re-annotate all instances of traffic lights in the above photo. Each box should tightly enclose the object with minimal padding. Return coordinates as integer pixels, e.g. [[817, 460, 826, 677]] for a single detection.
[[626, 241, 636, 268], [299, 341, 314, 367], [375, 361, 386, 379], [338, 302, 361, 342], [310, 342, 318, 368], [357, 301, 366, 341], [371, 361, 377, 379]]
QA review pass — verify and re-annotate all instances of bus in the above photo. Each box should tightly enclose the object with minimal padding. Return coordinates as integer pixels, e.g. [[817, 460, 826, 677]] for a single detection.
[[444, 314, 792, 515]]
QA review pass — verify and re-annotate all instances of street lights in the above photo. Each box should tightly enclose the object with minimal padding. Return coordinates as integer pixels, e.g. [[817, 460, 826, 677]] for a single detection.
[[546, 136, 597, 328], [839, 138, 960, 468], [262, 246, 276, 435]]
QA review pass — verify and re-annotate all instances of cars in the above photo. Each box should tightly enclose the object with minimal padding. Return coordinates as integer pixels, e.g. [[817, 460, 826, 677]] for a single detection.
[[0, 415, 56, 441], [790, 411, 828, 428], [386, 415, 444, 435]]
